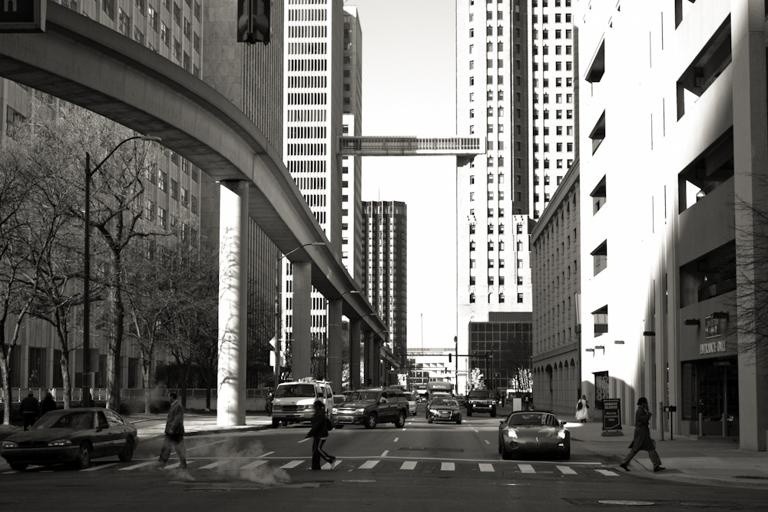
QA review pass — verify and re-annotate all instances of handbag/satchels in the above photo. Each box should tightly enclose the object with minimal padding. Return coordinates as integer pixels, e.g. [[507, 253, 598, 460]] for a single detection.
[[325, 416, 333, 432]]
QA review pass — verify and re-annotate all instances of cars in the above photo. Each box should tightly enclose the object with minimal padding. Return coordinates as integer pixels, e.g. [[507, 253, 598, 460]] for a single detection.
[[0, 406, 143, 474], [329, 380, 499, 429]]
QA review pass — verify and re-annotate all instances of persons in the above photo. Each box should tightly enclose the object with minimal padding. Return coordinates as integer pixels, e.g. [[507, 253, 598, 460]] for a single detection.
[[494, 391, 501, 407], [154, 391, 188, 469], [304, 399, 336, 471], [501, 392, 506, 408], [264, 387, 274, 416], [38, 391, 56, 417], [620, 396, 666, 471], [575, 394, 589, 423], [17, 391, 42, 429]]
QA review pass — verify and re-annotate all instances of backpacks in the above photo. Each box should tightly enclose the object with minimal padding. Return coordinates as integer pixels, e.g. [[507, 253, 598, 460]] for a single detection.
[[577, 402, 583, 412]]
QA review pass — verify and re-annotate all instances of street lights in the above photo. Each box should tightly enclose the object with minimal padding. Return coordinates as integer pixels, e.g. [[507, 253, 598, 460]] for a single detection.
[[323, 289, 362, 379], [348, 312, 380, 388], [81, 134, 162, 400], [274, 240, 329, 387]]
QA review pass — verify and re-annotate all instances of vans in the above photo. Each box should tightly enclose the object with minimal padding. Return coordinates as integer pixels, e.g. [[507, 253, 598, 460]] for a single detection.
[[269, 376, 336, 429]]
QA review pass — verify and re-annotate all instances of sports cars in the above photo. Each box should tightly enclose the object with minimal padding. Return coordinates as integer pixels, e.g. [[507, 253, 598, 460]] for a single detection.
[[496, 410, 572, 461]]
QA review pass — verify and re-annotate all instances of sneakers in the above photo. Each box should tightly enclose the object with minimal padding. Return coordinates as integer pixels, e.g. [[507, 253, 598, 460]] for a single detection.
[[307, 467, 313, 471], [619, 463, 631, 472], [653, 465, 666, 473], [330, 456, 337, 471]]
